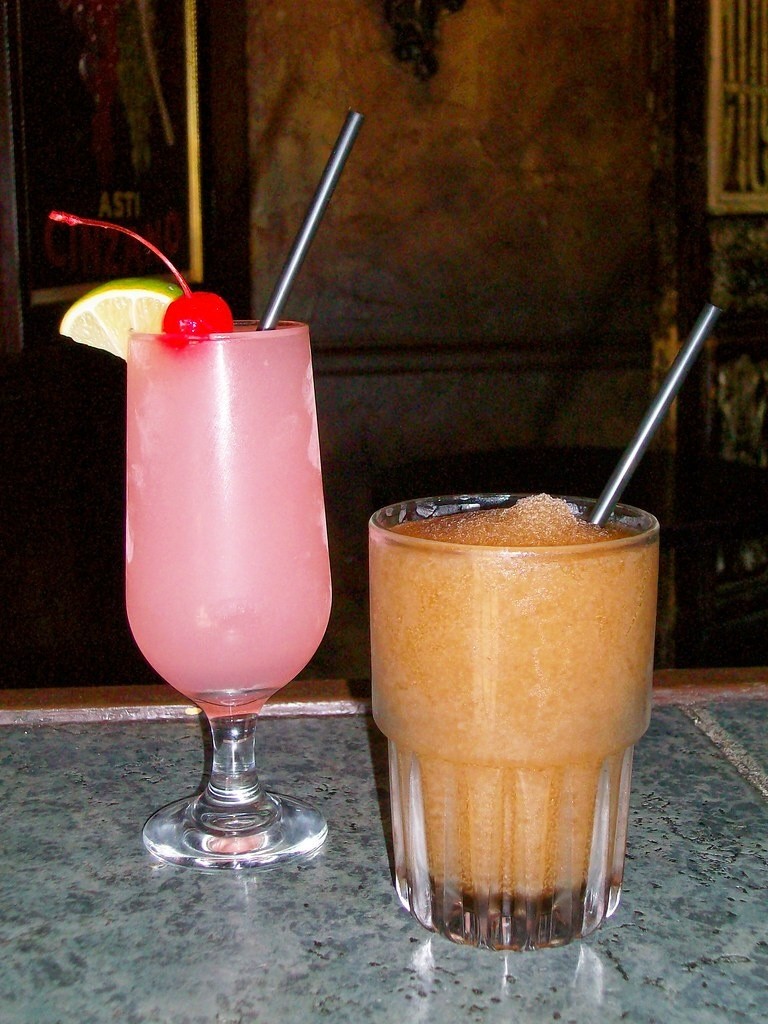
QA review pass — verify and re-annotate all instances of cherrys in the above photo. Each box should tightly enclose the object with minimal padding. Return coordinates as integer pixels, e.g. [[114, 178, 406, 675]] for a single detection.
[[50, 211, 233, 340]]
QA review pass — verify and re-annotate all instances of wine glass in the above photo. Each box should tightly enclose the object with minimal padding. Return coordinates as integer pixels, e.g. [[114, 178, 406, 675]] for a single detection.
[[123, 319, 333, 872]]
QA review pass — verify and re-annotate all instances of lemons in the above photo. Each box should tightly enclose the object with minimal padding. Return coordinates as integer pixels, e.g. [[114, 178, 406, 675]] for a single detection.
[[58, 277, 183, 362]]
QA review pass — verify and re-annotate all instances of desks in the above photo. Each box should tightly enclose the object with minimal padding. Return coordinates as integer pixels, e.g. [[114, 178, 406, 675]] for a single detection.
[[0, 665, 768, 1024]]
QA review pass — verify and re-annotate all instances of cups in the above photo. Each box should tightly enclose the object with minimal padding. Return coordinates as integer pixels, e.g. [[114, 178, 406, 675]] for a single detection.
[[367, 490, 662, 952]]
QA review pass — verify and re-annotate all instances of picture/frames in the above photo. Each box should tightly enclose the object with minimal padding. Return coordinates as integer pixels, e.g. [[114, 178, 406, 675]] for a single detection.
[[5, 0, 204, 310]]
